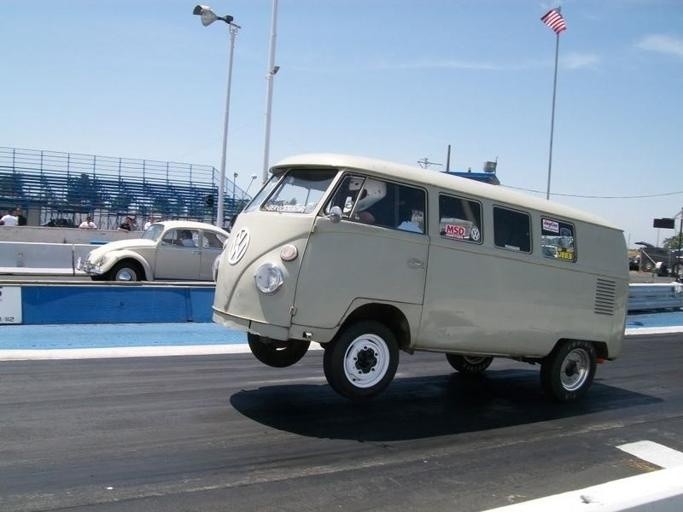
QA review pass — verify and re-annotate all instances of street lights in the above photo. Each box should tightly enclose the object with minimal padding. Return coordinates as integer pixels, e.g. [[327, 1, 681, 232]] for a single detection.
[[233, 171, 238, 208], [242, 175, 257, 202], [193, 5, 241, 227]]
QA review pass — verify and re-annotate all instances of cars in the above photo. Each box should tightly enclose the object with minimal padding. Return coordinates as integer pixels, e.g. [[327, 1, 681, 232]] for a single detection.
[[75, 220, 229, 282]]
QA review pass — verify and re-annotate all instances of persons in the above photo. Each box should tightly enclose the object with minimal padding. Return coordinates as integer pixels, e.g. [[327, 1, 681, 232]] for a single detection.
[[14, 207, 28, 226], [0, 207, 19, 227], [632, 254, 641, 271], [627, 255, 634, 270], [142, 215, 155, 233], [349, 191, 385, 226], [160, 229, 195, 248], [76, 216, 97, 229], [115, 216, 136, 233]]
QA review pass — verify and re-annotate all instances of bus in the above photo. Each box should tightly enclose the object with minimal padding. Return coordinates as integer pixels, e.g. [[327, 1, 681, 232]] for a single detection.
[[210, 153, 630, 401]]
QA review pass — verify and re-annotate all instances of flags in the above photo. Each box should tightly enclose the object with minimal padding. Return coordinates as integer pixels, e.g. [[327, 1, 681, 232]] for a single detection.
[[536, 6, 567, 35]]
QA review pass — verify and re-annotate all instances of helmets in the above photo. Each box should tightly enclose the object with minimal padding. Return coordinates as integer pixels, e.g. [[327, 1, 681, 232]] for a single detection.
[[348, 176, 388, 212]]
